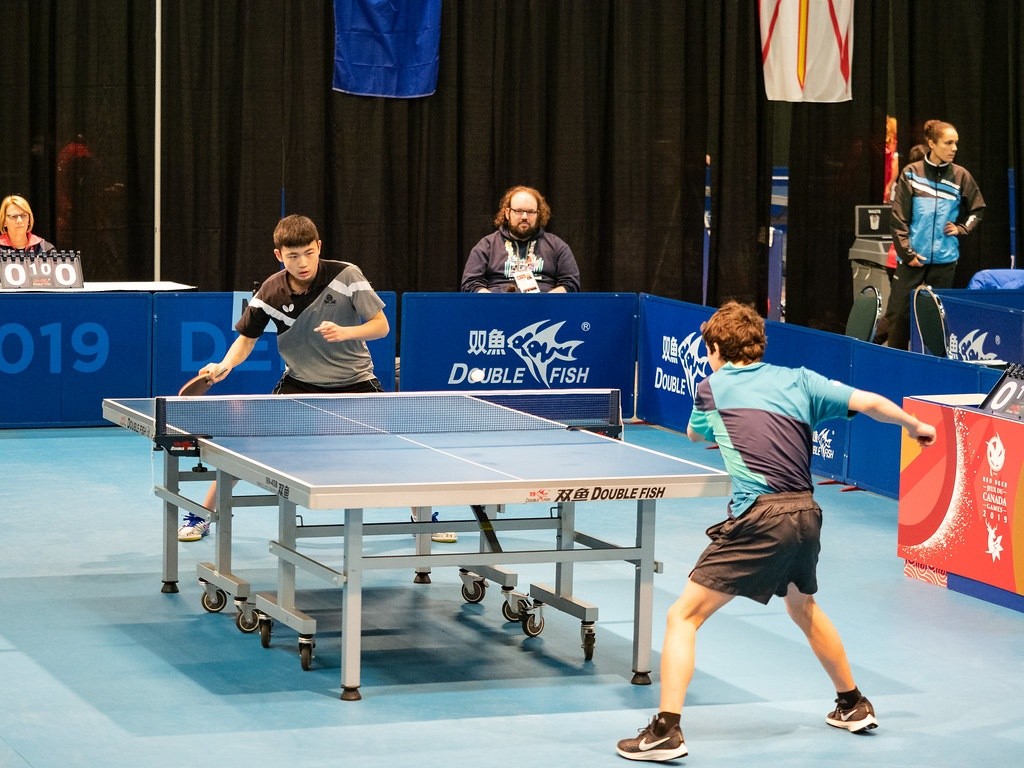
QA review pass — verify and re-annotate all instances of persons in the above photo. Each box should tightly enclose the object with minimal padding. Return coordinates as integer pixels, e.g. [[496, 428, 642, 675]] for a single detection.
[[0, 196, 57, 252], [462, 186, 581, 293], [616, 301, 937, 761], [872, 145, 930, 345], [885, 120, 986, 351], [173, 214, 462, 544]]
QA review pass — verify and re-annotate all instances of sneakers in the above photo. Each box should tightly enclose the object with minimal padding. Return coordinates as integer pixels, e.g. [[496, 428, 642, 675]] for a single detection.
[[411, 512, 458, 543], [178, 512, 211, 542], [826, 697, 878, 733], [616, 715, 688, 761]]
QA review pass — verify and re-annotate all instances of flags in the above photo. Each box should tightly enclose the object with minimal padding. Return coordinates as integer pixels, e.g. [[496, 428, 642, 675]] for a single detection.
[[332, 0, 442, 98], [760, 0, 854, 103]]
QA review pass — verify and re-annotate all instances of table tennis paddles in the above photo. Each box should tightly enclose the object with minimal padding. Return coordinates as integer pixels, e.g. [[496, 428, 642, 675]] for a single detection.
[[178, 366, 228, 396]]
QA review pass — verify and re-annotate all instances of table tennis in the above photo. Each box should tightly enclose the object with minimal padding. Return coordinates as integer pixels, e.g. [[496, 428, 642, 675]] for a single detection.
[[471, 370, 484, 382]]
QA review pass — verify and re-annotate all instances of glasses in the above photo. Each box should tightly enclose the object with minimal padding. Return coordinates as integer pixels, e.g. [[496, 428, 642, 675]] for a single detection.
[[511, 208, 538, 217], [6, 214, 28, 220]]
